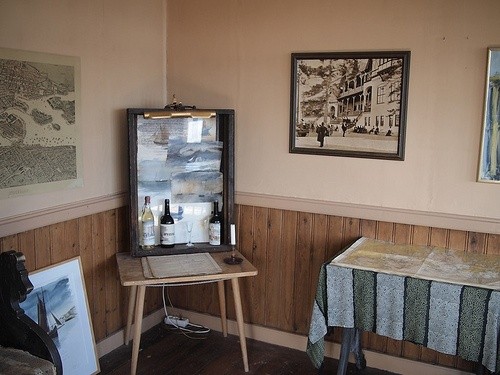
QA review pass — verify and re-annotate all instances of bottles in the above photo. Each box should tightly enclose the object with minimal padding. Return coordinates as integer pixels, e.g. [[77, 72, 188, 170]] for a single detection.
[[141, 196, 155, 250], [159, 199, 175, 248], [209, 200, 223, 246]]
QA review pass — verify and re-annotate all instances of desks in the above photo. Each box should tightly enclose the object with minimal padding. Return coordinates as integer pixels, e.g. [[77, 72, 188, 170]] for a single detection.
[[116, 246, 258, 375], [305, 236, 500, 375]]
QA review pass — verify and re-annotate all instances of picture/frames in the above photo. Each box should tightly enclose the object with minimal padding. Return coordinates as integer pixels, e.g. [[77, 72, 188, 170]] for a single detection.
[[475, 46, 500, 184], [289, 51, 412, 162], [17, 256, 102, 375]]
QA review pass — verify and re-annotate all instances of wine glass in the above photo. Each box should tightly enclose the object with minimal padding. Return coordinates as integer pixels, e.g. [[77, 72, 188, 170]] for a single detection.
[[184, 220, 194, 246]]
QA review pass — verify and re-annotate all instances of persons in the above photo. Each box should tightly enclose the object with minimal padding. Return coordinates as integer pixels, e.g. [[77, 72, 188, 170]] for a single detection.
[[386, 126, 392, 136], [368, 125, 380, 135], [315, 122, 329, 147], [342, 119, 347, 136]]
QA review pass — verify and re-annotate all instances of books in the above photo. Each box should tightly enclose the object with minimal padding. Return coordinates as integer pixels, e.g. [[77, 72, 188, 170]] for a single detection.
[[141, 252, 223, 279]]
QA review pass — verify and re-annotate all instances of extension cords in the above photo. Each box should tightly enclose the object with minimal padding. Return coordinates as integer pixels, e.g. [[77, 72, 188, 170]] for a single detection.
[[164, 315, 190, 327]]
